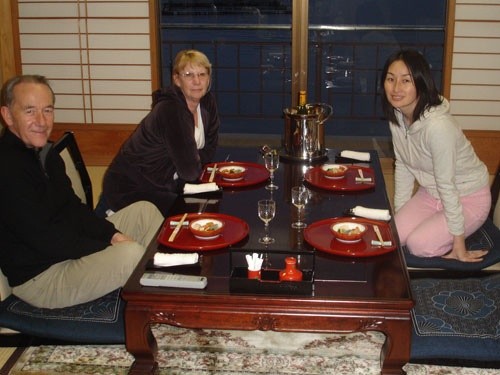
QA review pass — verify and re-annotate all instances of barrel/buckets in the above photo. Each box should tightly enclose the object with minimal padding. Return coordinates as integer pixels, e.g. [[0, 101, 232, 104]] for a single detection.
[[282, 102, 334, 158]]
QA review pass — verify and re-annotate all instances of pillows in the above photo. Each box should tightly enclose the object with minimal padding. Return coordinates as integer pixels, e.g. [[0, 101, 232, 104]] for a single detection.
[[409, 269, 500, 365], [402, 217, 500, 269], [0, 285, 126, 344]]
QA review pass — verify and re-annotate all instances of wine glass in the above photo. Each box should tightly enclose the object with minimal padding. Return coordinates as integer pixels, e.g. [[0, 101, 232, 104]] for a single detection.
[[305, 138, 317, 169], [258, 200, 275, 244], [264, 152, 279, 191], [291, 187, 309, 229]]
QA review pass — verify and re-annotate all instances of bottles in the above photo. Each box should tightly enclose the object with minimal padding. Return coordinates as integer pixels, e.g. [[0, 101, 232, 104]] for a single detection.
[[296, 90, 308, 114], [279, 257, 303, 282]]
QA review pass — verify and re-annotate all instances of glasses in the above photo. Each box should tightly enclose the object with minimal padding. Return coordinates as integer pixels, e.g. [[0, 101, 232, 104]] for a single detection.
[[176, 71, 210, 81]]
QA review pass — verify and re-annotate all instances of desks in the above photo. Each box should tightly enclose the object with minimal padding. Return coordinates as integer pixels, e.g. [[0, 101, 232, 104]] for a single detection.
[[119, 143, 416, 375]]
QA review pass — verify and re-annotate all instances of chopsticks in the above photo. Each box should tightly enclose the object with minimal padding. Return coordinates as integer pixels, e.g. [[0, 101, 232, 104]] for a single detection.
[[245, 253, 263, 271], [373, 224, 384, 247], [358, 169, 364, 180], [168, 213, 188, 242], [209, 163, 217, 182]]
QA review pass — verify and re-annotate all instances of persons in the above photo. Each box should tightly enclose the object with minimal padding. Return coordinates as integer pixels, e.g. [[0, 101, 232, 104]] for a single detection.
[[379, 49, 492, 263], [0, 74, 165, 310], [100, 50, 224, 218]]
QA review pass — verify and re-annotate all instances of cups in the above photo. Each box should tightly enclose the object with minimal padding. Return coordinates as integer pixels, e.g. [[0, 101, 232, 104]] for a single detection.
[[247, 268, 261, 280]]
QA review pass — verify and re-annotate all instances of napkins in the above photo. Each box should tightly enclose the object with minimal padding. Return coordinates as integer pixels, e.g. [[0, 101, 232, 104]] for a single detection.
[[340, 149, 372, 162], [352, 204, 392, 222], [182, 183, 220, 195], [152, 251, 199, 267]]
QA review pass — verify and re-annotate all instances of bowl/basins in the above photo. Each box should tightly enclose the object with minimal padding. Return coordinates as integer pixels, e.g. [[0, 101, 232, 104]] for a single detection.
[[320, 164, 348, 177], [329, 221, 368, 241], [189, 218, 224, 237], [219, 166, 248, 178]]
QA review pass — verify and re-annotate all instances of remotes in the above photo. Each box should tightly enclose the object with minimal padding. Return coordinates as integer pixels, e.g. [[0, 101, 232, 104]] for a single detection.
[[140, 273, 208, 290]]
